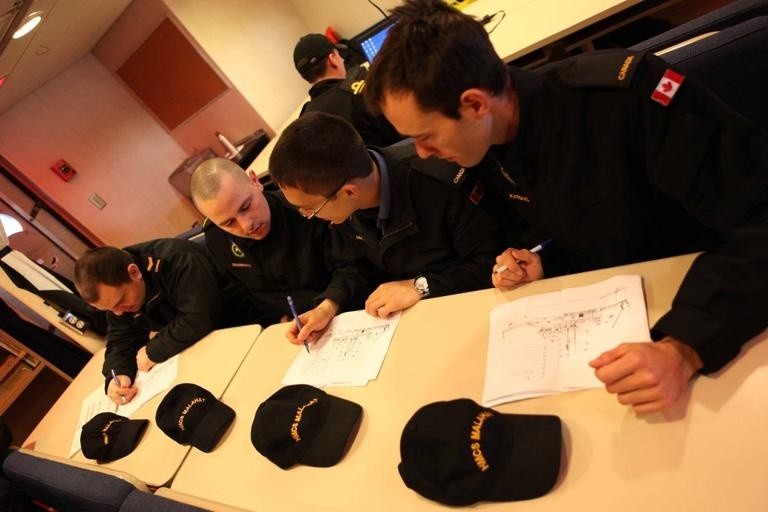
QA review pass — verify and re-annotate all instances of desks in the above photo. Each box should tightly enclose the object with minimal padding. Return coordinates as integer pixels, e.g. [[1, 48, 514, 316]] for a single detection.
[[243, 1, 768, 187], [188, 132, 270, 199], [20, 324, 265, 487], [170, 250, 768, 512]]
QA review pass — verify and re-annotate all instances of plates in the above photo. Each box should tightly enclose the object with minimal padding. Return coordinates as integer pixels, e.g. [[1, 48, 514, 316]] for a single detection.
[[224, 144, 245, 160]]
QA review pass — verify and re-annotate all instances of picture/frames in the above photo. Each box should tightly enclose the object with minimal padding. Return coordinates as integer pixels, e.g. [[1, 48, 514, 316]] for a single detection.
[[112, 11, 234, 138]]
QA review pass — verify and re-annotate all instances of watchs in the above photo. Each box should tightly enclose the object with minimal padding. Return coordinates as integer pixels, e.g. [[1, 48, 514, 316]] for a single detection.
[[413, 274, 431, 298]]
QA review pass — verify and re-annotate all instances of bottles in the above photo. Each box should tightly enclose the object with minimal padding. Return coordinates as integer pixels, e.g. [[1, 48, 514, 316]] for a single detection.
[[215, 131, 238, 155]]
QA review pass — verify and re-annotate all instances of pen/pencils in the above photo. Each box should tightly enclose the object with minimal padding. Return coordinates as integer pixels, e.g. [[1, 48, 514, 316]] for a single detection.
[[494, 239, 552, 274], [287, 296, 310, 355], [110, 368, 125, 401]]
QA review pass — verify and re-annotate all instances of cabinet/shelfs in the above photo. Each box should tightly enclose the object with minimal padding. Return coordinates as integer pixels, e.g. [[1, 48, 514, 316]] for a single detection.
[[0, 297, 74, 467]]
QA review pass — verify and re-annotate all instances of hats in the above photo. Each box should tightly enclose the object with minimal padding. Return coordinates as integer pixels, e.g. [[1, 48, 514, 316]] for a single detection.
[[156, 383, 235, 452], [398, 399, 561, 506], [251, 384, 362, 469], [81, 412, 148, 461], [294, 34, 348, 72]]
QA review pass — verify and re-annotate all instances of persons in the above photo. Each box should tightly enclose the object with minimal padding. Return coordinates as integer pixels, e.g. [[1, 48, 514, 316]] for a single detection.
[[294, 34, 408, 148], [73, 240, 257, 399], [192, 158, 337, 326], [269, 111, 502, 347], [363, 0, 768, 414]]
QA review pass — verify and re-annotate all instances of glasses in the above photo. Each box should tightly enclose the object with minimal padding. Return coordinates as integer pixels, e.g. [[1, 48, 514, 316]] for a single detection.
[[295, 180, 346, 220]]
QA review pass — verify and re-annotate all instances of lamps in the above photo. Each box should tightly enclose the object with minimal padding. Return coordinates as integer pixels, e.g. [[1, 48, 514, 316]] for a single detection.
[[12, 11, 44, 40]]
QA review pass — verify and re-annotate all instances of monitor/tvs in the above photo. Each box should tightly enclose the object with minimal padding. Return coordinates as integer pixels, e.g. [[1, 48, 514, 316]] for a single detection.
[[347, 14, 397, 66]]
